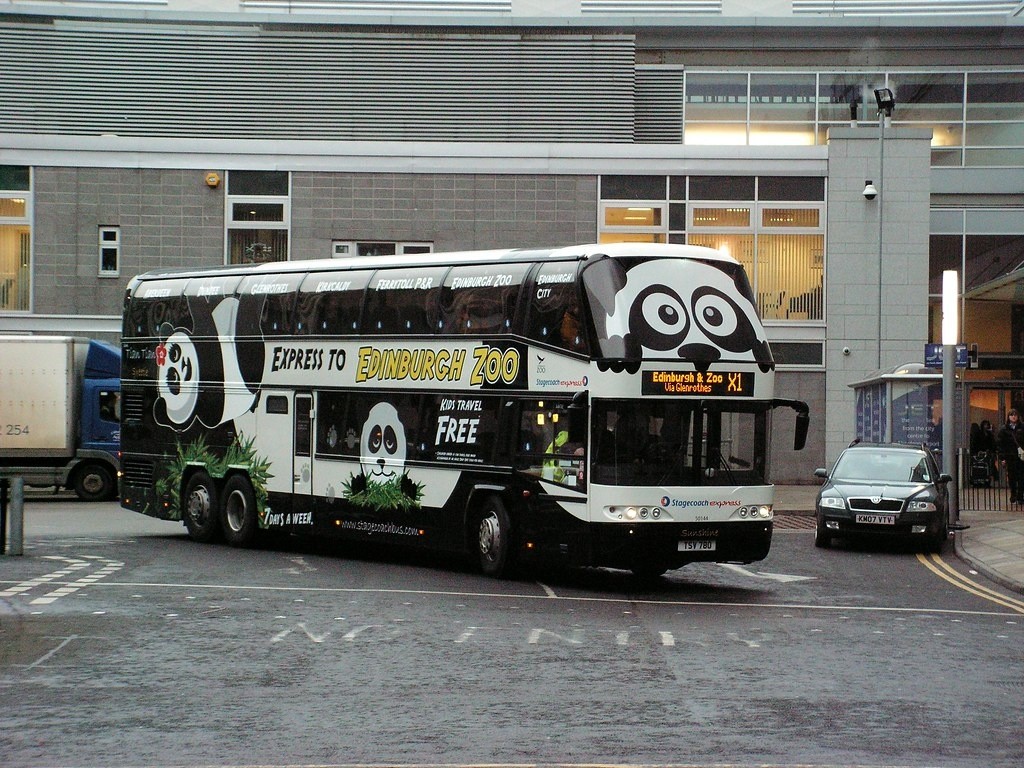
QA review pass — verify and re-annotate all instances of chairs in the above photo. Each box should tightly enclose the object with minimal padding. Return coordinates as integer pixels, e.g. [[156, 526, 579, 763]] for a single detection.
[[273, 305, 581, 352], [763, 291, 786, 317], [138, 322, 161, 338]]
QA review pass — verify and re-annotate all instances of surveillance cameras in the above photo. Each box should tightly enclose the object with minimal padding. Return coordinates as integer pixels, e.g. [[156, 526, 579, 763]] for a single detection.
[[863, 185, 878, 201], [843, 346, 851, 355]]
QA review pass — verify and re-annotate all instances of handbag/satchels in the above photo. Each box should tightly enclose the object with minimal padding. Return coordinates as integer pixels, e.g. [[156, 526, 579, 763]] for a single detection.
[[1017, 447, 1024, 461]]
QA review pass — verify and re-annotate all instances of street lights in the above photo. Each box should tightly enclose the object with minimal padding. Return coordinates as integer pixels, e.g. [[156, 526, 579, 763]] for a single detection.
[[941, 269, 972, 529]]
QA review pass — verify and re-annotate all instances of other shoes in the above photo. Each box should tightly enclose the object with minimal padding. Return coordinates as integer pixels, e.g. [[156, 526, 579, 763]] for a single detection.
[[1010, 495, 1017, 503], [1018, 496, 1024, 505]]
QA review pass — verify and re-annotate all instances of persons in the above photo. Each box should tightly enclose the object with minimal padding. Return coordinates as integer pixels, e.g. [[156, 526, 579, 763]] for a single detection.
[[539, 430, 584, 490], [927, 416, 996, 488], [997, 408, 1024, 503], [100, 394, 119, 423]]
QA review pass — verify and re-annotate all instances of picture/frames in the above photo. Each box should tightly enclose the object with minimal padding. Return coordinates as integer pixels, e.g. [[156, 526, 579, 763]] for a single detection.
[[810, 248, 824, 270], [740, 240, 770, 264]]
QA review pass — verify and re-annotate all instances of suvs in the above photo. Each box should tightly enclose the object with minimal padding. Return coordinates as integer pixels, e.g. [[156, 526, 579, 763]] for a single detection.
[[814, 439, 953, 551]]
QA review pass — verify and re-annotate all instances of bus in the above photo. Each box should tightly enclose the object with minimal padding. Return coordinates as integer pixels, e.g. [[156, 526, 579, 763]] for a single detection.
[[120, 243, 810, 579]]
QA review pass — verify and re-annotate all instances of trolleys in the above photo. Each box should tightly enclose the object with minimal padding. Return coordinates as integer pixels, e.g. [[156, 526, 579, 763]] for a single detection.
[[971, 452, 993, 486]]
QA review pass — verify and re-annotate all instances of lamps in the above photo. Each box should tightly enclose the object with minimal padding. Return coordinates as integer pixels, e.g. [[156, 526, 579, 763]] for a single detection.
[[862, 181, 877, 200], [874, 87, 895, 117]]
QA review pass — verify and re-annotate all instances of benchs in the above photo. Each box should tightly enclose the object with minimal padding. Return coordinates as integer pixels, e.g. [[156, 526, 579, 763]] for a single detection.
[[787, 296, 815, 317]]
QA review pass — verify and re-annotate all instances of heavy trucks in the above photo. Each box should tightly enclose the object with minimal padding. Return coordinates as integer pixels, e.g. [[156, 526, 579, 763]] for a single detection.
[[0, 334, 120, 501]]
